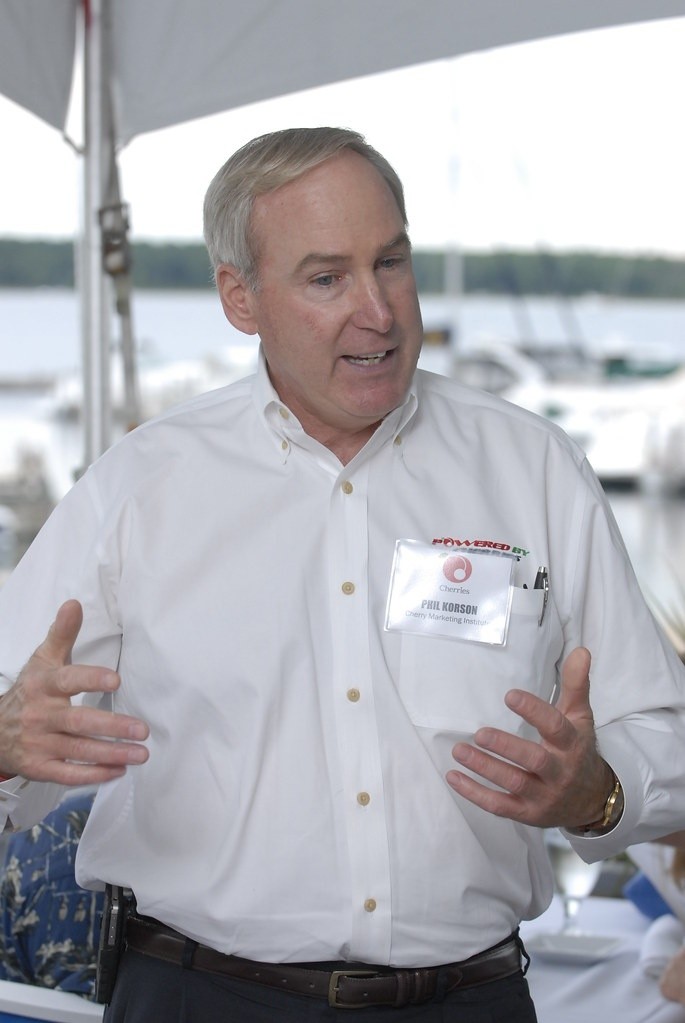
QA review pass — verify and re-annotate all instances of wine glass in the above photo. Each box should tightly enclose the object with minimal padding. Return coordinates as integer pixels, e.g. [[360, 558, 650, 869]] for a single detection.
[[538, 820, 604, 931]]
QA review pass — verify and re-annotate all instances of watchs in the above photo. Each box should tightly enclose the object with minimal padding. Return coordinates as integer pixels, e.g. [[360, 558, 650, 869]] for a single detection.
[[569, 771, 624, 832]]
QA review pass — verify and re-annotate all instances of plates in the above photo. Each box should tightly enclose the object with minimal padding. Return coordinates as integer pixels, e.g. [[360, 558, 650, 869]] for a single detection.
[[522, 933, 630, 964]]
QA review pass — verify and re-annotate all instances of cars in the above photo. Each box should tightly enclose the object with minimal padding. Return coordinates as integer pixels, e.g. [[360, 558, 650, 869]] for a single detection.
[[410, 339, 664, 491]]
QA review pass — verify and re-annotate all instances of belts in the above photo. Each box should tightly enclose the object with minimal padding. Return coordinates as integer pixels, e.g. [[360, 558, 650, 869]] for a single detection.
[[119, 900, 522, 1012]]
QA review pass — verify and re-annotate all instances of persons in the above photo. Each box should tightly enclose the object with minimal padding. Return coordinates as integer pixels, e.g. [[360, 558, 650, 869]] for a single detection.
[[0, 126, 685, 1023]]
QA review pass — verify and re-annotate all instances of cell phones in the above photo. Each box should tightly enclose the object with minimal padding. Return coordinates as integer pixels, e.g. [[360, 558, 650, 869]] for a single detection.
[[94, 881, 124, 1004]]
